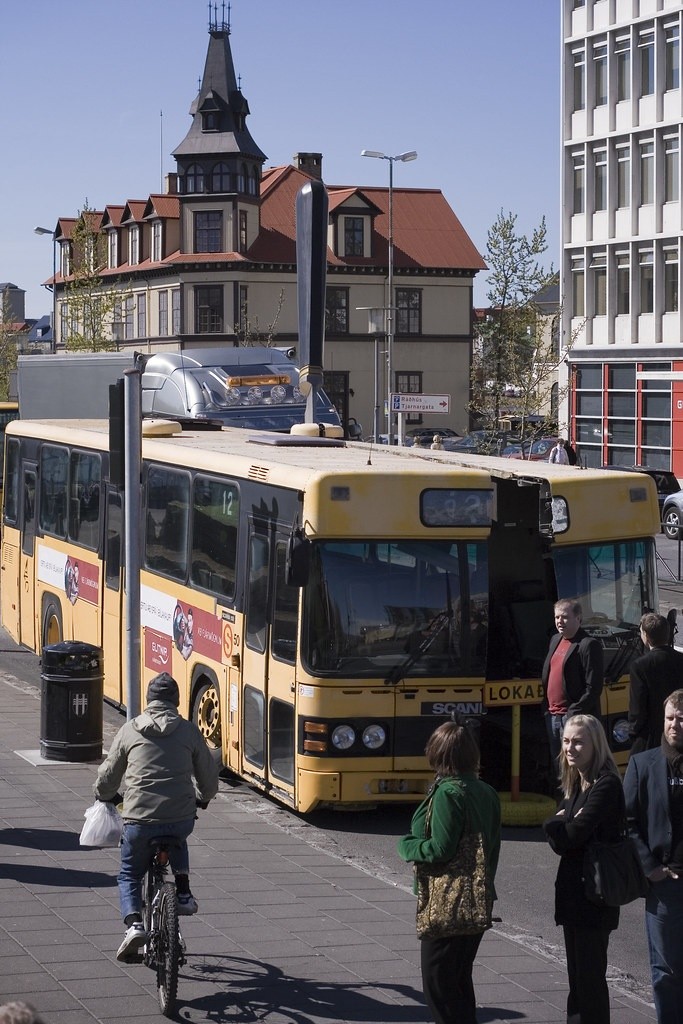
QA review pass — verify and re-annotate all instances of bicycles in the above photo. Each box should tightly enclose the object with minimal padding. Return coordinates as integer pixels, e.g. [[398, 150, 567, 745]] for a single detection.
[[96, 795, 194, 1017]]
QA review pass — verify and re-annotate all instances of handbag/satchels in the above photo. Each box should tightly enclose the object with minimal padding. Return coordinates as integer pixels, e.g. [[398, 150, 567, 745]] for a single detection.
[[412, 797, 493, 940], [587, 774, 645, 906], [79, 799, 122, 847]]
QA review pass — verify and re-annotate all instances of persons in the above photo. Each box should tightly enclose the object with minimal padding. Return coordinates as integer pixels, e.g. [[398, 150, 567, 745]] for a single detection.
[[540, 598, 603, 806], [411, 436, 421, 448], [623, 689, 683, 1024], [430, 435, 445, 450], [627, 613, 683, 759], [65, 561, 79, 598], [175, 609, 193, 659], [549, 439, 569, 465], [397, 721, 502, 1024], [91, 672, 218, 961], [563, 440, 577, 465], [544, 714, 624, 1024]]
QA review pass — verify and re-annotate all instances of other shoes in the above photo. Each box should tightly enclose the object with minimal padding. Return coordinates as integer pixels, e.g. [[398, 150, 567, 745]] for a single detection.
[[116, 921, 146, 962], [177, 895, 198, 915]]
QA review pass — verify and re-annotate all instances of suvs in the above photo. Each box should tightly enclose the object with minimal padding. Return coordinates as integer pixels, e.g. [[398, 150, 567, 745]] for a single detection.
[[596, 465, 680, 521]]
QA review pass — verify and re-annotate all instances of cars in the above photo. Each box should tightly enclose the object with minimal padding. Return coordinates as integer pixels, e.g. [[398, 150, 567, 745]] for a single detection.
[[365, 426, 566, 463], [661, 487, 683, 540]]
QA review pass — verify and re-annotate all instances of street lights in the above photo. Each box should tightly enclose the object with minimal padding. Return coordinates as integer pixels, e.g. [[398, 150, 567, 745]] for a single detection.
[[355, 306, 400, 444], [359, 148, 418, 447], [32, 227, 57, 353]]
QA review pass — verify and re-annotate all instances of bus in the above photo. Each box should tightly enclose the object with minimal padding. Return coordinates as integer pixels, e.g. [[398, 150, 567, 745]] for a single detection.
[[0, 417, 681, 830], [0, 401, 20, 541]]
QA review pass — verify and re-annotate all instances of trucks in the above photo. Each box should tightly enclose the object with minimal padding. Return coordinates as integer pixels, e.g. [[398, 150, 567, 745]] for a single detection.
[[13, 347, 365, 444]]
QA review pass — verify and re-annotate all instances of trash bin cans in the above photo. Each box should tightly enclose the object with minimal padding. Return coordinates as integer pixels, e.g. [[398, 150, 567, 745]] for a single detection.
[[40, 640, 105, 762]]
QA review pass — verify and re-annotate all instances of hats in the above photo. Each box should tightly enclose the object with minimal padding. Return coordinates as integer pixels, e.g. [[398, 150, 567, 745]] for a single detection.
[[147, 672, 179, 705], [433, 434, 443, 443]]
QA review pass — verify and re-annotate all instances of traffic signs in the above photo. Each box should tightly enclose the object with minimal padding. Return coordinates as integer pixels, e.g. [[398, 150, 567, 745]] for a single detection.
[[389, 392, 452, 414]]
